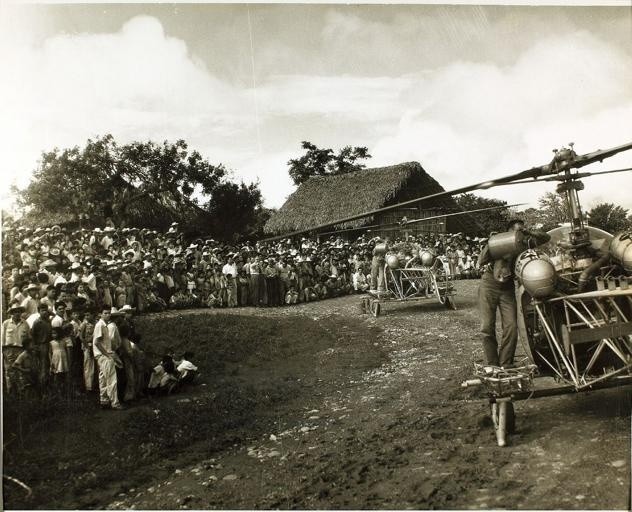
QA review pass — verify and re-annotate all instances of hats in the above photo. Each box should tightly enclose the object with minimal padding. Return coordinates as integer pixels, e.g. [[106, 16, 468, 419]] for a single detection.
[[170, 222, 179, 226], [3, 226, 157, 239], [110, 305, 136, 316], [24, 284, 40, 291], [7, 303, 26, 313], [49, 248, 60, 256], [186, 236, 363, 260]]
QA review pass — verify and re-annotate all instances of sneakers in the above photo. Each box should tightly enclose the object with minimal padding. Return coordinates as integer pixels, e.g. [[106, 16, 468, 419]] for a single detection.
[[114, 405, 126, 410]]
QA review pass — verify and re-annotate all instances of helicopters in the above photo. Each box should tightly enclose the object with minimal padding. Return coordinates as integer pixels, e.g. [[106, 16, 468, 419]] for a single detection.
[[265, 140, 632, 448], [316, 201, 529, 318]]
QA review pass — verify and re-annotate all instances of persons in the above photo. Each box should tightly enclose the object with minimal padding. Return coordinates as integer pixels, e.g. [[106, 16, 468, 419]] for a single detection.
[[478, 219, 548, 373], [2, 218, 487, 410]]
